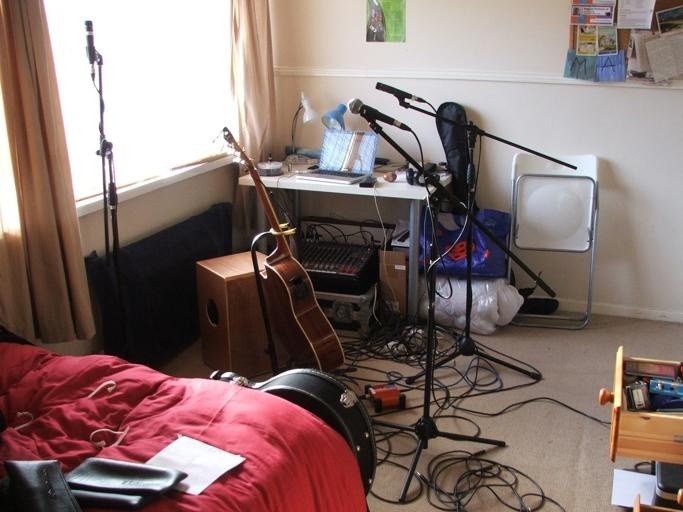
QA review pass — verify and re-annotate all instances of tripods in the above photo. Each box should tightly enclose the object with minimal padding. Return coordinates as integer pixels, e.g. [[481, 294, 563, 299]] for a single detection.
[[369, 131, 504, 502], [405, 109, 542, 387]]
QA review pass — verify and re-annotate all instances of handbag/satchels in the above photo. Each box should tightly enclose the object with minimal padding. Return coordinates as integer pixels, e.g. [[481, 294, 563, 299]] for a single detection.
[[0, 456, 188, 511], [419, 204, 510, 279]]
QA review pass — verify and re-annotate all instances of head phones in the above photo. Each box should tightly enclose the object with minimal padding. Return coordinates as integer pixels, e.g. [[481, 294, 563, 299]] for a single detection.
[[407, 166, 421, 185]]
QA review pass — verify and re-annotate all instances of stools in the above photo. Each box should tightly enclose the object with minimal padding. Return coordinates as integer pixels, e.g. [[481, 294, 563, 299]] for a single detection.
[[195, 250, 287, 378]]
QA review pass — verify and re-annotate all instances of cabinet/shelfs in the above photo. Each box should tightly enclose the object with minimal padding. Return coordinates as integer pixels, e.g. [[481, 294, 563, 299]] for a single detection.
[[597, 343, 683, 511]]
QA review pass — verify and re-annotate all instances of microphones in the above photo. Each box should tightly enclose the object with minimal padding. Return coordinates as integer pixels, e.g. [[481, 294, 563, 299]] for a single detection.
[[348, 98, 409, 131], [376, 80, 428, 104], [85, 20, 96, 78], [298, 129, 379, 186]]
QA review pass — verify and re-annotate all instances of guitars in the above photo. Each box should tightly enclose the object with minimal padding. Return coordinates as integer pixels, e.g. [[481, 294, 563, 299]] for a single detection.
[[222, 127, 346, 371]]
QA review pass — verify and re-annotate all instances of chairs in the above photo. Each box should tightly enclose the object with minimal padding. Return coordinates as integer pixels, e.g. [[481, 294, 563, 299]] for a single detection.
[[506, 153, 600, 330]]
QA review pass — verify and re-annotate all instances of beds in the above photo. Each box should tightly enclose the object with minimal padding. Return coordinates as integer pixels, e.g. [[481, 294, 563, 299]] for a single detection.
[[0, 324, 367, 512]]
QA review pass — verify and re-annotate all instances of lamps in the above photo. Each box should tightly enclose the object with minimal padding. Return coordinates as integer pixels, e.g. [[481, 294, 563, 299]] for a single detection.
[[320, 102, 347, 131], [285, 96, 316, 164]]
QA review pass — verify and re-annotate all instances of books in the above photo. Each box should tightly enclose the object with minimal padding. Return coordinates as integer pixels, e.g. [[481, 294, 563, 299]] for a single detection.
[[648, 394, 683, 413], [625, 361, 675, 380]]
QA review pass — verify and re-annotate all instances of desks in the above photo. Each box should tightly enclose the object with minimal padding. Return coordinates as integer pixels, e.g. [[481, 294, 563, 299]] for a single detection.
[[238, 156, 452, 322]]
